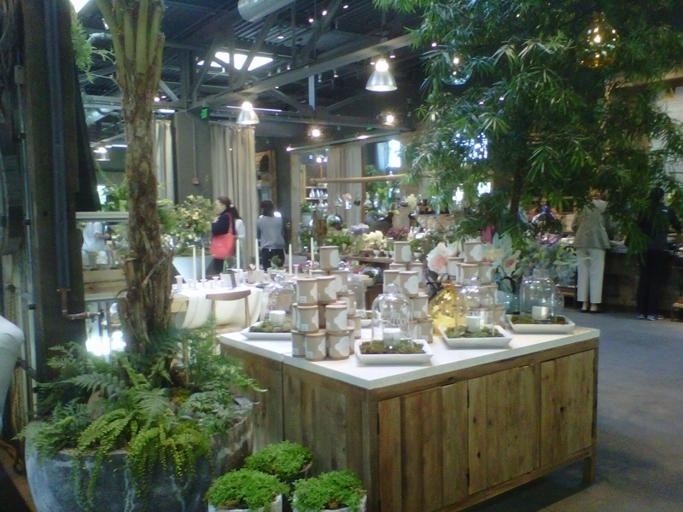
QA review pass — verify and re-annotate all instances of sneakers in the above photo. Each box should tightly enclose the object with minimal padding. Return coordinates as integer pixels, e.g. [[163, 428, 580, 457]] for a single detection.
[[637, 311, 664, 321]]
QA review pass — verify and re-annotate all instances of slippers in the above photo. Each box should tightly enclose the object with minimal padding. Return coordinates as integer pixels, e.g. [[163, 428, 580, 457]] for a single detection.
[[580, 305, 608, 313]]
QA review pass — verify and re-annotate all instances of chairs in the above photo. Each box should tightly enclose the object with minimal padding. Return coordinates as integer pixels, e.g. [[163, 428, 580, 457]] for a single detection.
[[198, 290, 251, 340]]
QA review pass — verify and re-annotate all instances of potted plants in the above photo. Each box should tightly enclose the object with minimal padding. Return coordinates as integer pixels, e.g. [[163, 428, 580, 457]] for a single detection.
[[243, 440, 314, 491], [290, 469, 369, 512], [204, 468, 291, 512], [10, 307, 270, 512]]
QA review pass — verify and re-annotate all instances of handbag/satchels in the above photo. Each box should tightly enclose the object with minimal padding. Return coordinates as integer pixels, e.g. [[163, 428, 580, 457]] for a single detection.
[[209, 211, 236, 261]]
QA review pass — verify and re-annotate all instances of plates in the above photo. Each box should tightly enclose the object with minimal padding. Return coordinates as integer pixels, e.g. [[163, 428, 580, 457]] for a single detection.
[[356, 309, 381, 328], [506, 311, 576, 335], [355, 337, 434, 364], [245, 319, 292, 341], [438, 323, 512, 349]]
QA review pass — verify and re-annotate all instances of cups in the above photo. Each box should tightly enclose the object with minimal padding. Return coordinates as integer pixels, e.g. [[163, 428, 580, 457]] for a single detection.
[[269, 309, 287, 325], [465, 314, 481, 333], [381, 328, 400, 347], [532, 306, 550, 322]]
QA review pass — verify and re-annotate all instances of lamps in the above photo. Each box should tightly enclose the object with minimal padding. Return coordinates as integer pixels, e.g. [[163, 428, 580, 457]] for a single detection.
[[92, 143, 107, 153], [192, 238, 316, 287], [94, 153, 110, 161], [437, 38, 473, 86], [365, 45, 398, 92], [576, 0, 622, 71], [235, 92, 260, 125]]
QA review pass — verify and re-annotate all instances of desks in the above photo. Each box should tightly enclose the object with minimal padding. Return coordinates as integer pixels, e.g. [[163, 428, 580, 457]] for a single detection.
[[110, 278, 264, 335], [238, 268, 370, 318]]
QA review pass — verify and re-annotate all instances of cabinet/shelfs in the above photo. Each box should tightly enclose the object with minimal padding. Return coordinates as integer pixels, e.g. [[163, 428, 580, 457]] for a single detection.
[[214, 309, 601, 512], [305, 186, 328, 211], [552, 248, 683, 318]]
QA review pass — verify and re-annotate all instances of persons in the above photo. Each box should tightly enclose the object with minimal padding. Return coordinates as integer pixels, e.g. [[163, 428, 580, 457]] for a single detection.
[[570, 188, 621, 313], [256, 199, 288, 272], [206, 196, 241, 277], [625, 187, 682, 320], [0, 313, 35, 510]]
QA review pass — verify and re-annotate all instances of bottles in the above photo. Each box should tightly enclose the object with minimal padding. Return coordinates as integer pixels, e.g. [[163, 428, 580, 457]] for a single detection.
[[308, 188, 328, 211], [442, 238, 505, 328], [366, 188, 399, 212], [292, 244, 362, 360], [381, 239, 432, 338]]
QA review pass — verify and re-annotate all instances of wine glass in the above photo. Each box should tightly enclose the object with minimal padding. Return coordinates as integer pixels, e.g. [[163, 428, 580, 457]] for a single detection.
[[175, 259, 291, 302], [373, 250, 381, 258]]
